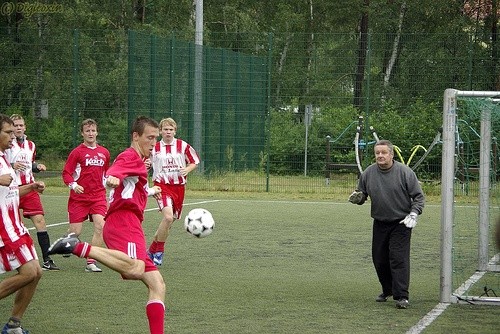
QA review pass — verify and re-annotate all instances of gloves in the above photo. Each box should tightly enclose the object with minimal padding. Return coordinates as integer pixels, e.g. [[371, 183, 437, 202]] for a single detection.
[[348, 192, 363, 203], [399, 211, 417, 228]]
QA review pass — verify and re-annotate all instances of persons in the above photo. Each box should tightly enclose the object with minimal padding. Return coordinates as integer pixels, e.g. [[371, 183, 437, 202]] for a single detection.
[[4, 113, 61, 272], [48, 114, 166, 334], [0, 113, 42, 334], [142, 119, 201, 267], [62, 118, 111, 273], [347, 140, 424, 308]]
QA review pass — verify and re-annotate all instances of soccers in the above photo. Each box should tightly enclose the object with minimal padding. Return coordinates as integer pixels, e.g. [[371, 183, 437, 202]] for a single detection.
[[184, 208, 215, 238]]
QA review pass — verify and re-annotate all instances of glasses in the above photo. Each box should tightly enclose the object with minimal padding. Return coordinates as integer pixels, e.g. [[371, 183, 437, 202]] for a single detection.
[[1, 129, 14, 136]]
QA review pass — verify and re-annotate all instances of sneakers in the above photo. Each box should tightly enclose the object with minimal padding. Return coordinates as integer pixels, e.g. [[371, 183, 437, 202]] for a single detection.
[[152, 252, 162, 266], [376, 293, 393, 302], [1, 323, 29, 334], [85, 264, 102, 272], [396, 299, 408, 309], [42, 260, 60, 272], [145, 249, 154, 260], [47, 233, 81, 255]]
